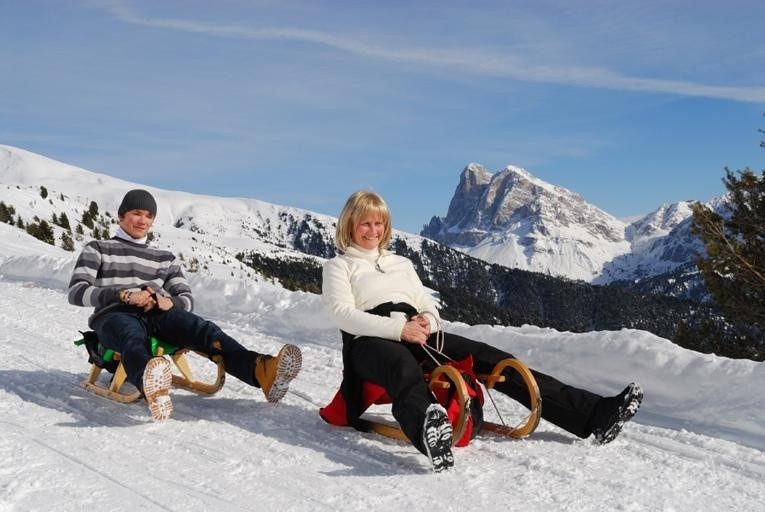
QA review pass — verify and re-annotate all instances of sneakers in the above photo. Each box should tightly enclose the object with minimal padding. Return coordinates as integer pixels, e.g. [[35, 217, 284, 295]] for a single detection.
[[421, 404, 454, 475], [588, 383, 643, 447]]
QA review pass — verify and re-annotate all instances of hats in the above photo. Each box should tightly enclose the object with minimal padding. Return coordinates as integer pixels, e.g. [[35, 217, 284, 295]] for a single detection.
[[118, 190, 157, 218]]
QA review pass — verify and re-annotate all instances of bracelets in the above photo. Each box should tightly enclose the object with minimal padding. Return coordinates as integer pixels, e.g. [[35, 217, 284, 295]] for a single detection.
[[121, 290, 129, 301], [125, 291, 134, 305]]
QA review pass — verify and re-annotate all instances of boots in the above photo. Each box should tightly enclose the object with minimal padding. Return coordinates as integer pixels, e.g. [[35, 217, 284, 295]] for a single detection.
[[140, 356, 174, 422], [254, 344, 302, 404]]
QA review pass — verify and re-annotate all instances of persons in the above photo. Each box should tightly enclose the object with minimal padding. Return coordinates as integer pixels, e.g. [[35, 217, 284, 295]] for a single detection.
[[320, 188, 645, 473], [65, 189, 303, 419]]
